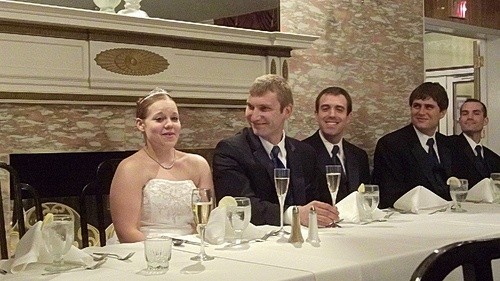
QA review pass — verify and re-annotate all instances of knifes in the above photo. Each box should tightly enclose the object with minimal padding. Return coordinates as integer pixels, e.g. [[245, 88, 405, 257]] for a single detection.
[[172, 237, 208, 245]]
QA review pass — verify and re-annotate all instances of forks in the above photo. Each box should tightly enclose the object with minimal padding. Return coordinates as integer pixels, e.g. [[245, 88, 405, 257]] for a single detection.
[[430, 208, 447, 215], [256, 234, 269, 242], [335, 218, 344, 223], [362, 212, 393, 225], [86, 261, 105, 270], [93, 252, 134, 260], [89, 253, 109, 261]]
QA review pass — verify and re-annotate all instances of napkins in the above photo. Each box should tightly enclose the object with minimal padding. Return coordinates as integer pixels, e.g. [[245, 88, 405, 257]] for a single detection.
[[10, 220, 95, 274], [204, 178, 500, 244]]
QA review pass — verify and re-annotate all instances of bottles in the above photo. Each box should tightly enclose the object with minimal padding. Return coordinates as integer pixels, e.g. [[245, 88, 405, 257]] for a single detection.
[[288, 207, 305, 243], [306, 206, 321, 243]]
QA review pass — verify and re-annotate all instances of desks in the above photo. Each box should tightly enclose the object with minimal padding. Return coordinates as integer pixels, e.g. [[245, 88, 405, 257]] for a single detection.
[[0, 199, 500, 281]]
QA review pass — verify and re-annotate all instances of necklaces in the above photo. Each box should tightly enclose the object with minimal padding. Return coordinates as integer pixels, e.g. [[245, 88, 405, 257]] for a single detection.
[[143, 148, 176, 169]]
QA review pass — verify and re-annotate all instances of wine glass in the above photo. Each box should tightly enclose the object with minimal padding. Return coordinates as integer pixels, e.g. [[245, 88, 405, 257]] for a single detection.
[[269, 168, 291, 235], [491, 173, 500, 204], [326, 165, 344, 228], [450, 179, 468, 213], [42, 214, 74, 272], [360, 185, 379, 223], [230, 197, 252, 249], [190, 188, 214, 261]]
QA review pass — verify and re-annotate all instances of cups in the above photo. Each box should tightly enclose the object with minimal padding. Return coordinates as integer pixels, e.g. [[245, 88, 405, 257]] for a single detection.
[[144, 238, 172, 270]]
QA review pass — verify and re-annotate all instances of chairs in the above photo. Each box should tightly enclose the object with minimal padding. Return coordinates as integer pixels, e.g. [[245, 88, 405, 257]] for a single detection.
[[408, 236, 500, 281], [79, 157, 123, 249], [0, 162, 43, 259]]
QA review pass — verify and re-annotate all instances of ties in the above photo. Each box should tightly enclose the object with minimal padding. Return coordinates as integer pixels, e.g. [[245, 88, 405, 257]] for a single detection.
[[331, 145, 346, 186], [426, 138, 440, 168], [271, 146, 287, 194], [475, 145, 483, 163]]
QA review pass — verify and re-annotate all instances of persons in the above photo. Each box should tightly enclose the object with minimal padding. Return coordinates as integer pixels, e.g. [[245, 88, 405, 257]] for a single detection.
[[106, 88, 215, 246], [448, 99, 500, 178], [301, 87, 370, 206], [213, 74, 339, 228], [374, 82, 483, 209]]
